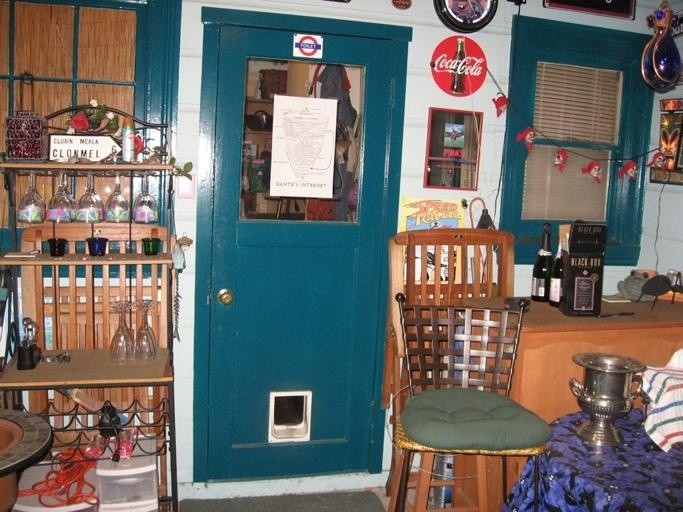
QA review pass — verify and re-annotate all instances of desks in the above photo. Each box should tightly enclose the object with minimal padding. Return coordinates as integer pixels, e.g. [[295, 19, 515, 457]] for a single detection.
[[494, 406, 683, 511]]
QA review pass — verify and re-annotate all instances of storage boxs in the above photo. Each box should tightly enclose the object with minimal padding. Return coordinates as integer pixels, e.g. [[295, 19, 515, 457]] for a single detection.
[[95, 432, 161, 512]]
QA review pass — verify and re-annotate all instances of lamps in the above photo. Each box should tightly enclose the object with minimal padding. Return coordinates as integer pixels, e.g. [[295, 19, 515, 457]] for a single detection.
[[468, 198, 498, 283]]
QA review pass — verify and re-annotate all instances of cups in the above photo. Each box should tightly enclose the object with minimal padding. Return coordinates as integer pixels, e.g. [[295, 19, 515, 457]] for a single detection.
[[141, 237, 160, 256], [85, 236, 108, 256], [46, 237, 67, 257]]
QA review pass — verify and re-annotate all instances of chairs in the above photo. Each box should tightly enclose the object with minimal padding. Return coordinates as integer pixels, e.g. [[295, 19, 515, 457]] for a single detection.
[[379, 226, 518, 494], [19, 223, 177, 497], [389, 292, 554, 512]]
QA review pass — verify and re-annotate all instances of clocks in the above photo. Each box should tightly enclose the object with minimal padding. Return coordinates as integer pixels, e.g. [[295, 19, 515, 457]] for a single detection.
[[431, 0, 498, 35]]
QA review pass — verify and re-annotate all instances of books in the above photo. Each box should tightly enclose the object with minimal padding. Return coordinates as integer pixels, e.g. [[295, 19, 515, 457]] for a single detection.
[[101, 505, 157, 512], [97, 472, 157, 507], [95, 435, 157, 475]]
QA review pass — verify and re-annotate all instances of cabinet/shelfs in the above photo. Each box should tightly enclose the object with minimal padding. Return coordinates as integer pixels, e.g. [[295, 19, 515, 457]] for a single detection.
[[461, 295, 683, 512], [1, 105, 184, 512]]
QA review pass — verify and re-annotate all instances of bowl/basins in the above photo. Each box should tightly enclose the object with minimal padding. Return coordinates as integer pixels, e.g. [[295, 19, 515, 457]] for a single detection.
[[246, 110, 272, 132]]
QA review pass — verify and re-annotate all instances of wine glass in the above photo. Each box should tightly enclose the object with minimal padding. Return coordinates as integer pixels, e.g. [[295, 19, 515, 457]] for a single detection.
[[569, 352, 648, 449], [109, 300, 157, 362]]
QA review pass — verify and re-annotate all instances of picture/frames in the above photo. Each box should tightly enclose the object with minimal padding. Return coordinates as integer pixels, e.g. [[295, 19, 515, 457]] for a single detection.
[[542, 0, 636, 21], [422, 106, 484, 194]]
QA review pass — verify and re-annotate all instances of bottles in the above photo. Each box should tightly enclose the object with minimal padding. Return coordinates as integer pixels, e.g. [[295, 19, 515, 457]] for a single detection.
[[529, 222, 554, 302], [450, 38, 467, 94], [546, 235, 563, 307], [83, 434, 108, 461], [118, 429, 132, 463], [16, 173, 160, 224], [122, 117, 135, 162]]
